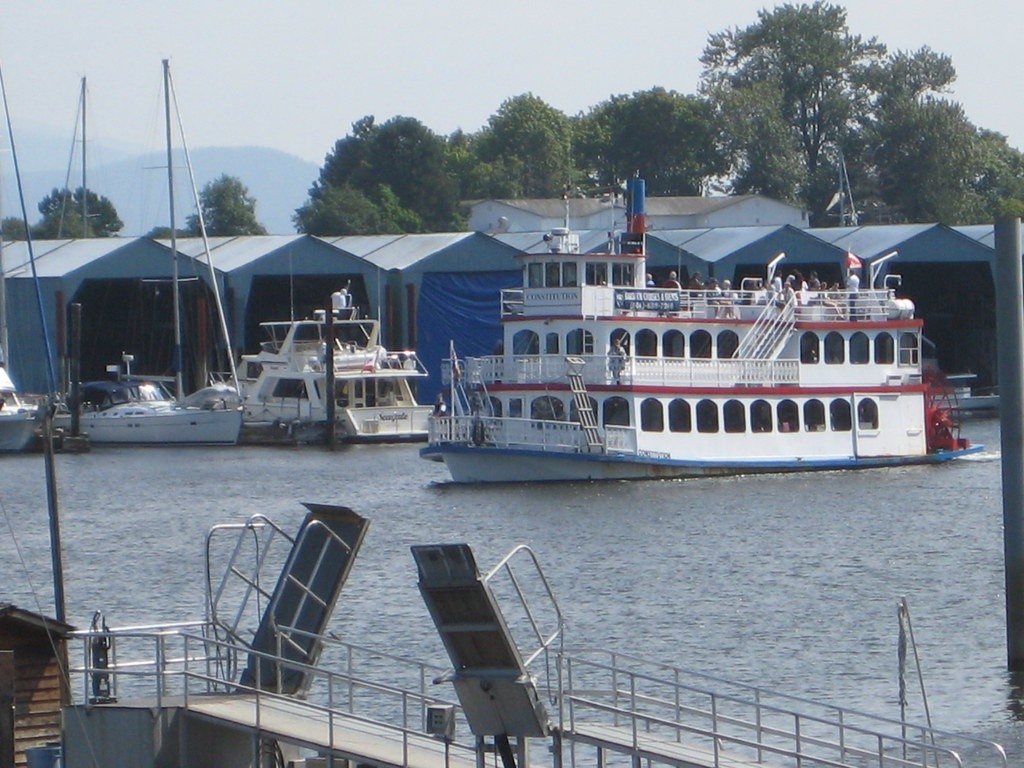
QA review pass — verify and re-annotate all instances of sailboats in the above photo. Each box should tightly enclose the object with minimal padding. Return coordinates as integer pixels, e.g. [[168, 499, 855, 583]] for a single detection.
[[1, 68, 57, 451], [46, 60, 243, 448]]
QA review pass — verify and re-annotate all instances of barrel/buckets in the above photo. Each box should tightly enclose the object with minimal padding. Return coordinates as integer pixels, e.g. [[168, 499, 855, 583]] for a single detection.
[[24, 746, 63, 766]]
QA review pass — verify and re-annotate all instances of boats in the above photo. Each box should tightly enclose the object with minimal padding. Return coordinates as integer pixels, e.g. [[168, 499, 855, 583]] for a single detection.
[[420, 178, 980, 482], [232, 279, 432, 444]]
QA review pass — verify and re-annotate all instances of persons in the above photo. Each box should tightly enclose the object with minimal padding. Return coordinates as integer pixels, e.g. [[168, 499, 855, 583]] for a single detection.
[[608, 339, 628, 386], [641, 269, 848, 319]]
